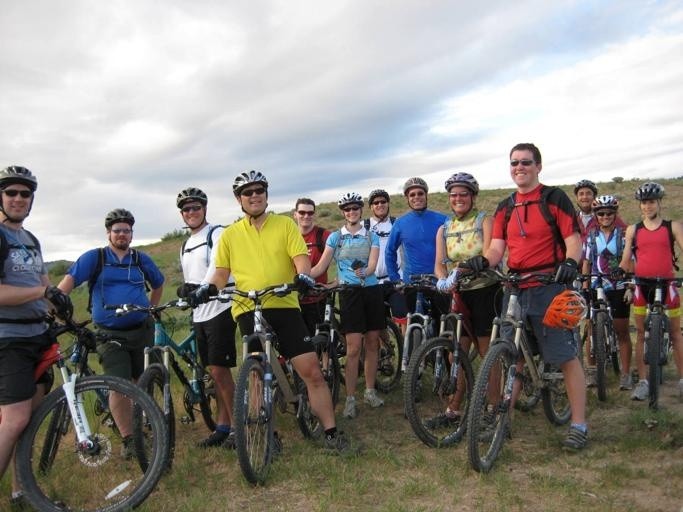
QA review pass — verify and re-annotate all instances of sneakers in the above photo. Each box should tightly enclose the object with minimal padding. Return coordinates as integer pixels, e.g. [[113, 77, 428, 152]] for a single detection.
[[479, 421, 513, 442], [423, 407, 462, 429], [631, 379, 651, 401], [560, 424, 589, 452], [364, 389, 385, 408], [344, 396, 356, 420], [586, 374, 599, 387], [619, 374, 633, 390], [223, 429, 250, 449], [324, 431, 351, 452], [196, 426, 229, 449], [121, 436, 140, 460], [266, 433, 282, 453], [11, 492, 67, 511]]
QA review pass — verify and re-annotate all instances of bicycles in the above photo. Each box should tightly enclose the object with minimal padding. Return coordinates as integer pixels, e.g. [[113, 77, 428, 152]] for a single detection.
[[104, 295, 233, 478], [196, 284, 340, 486], [388, 280, 478, 420], [295, 279, 402, 441], [457, 260, 583, 473], [612, 272, 683, 412], [574, 273, 635, 403], [34, 313, 123, 477], [402, 269, 542, 450], [0, 290, 170, 512]]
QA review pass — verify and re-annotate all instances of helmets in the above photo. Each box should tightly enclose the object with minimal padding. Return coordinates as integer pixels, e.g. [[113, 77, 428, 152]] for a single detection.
[[591, 196, 618, 211], [338, 192, 364, 209], [543, 291, 588, 331], [233, 169, 269, 195], [369, 189, 390, 207], [1, 167, 38, 191], [177, 188, 208, 209], [575, 180, 598, 196], [105, 209, 136, 227], [635, 183, 665, 201], [403, 178, 429, 195], [445, 173, 480, 196]]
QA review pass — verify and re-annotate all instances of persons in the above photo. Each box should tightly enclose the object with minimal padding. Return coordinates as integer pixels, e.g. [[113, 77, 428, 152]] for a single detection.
[[56, 207, 166, 464], [293, 198, 334, 377], [422, 173, 504, 429], [467, 143, 588, 456], [582, 195, 634, 390], [192, 170, 360, 458], [619, 182, 683, 401], [574, 179, 629, 233], [310, 192, 385, 420], [0, 165, 66, 511], [358, 183, 409, 344], [176, 187, 249, 451], [386, 178, 449, 322]]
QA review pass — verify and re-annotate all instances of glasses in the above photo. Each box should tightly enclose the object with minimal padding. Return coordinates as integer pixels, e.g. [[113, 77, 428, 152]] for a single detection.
[[111, 228, 133, 234], [2, 189, 34, 199], [181, 206, 203, 212], [240, 188, 267, 197], [344, 206, 363, 212], [410, 192, 425, 197], [298, 210, 316, 217], [511, 160, 537, 167], [371, 200, 388, 206], [449, 192, 469, 198], [597, 212, 615, 217]]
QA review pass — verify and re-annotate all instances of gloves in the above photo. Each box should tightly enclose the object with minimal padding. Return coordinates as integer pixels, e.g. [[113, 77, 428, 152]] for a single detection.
[[177, 283, 198, 297], [466, 255, 490, 273], [446, 270, 464, 289], [436, 278, 458, 295], [611, 268, 625, 281], [555, 259, 577, 286], [191, 285, 218, 306], [45, 285, 72, 319], [294, 274, 316, 291]]
